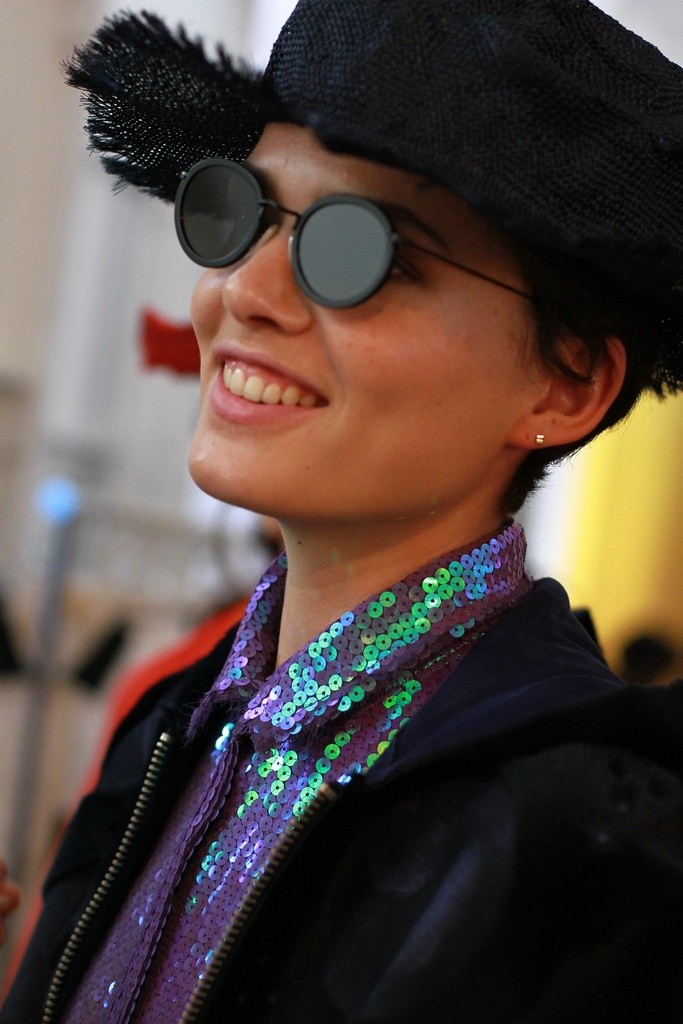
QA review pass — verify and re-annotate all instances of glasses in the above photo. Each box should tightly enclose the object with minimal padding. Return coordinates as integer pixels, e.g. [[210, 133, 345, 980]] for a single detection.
[[174, 158, 550, 311]]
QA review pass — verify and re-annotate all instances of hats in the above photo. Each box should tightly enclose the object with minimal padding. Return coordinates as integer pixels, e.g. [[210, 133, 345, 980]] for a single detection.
[[66, 1, 683, 401]]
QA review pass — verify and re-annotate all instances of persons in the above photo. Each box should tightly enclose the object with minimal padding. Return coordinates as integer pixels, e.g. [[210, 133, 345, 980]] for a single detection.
[[0, 0, 683, 1024]]
